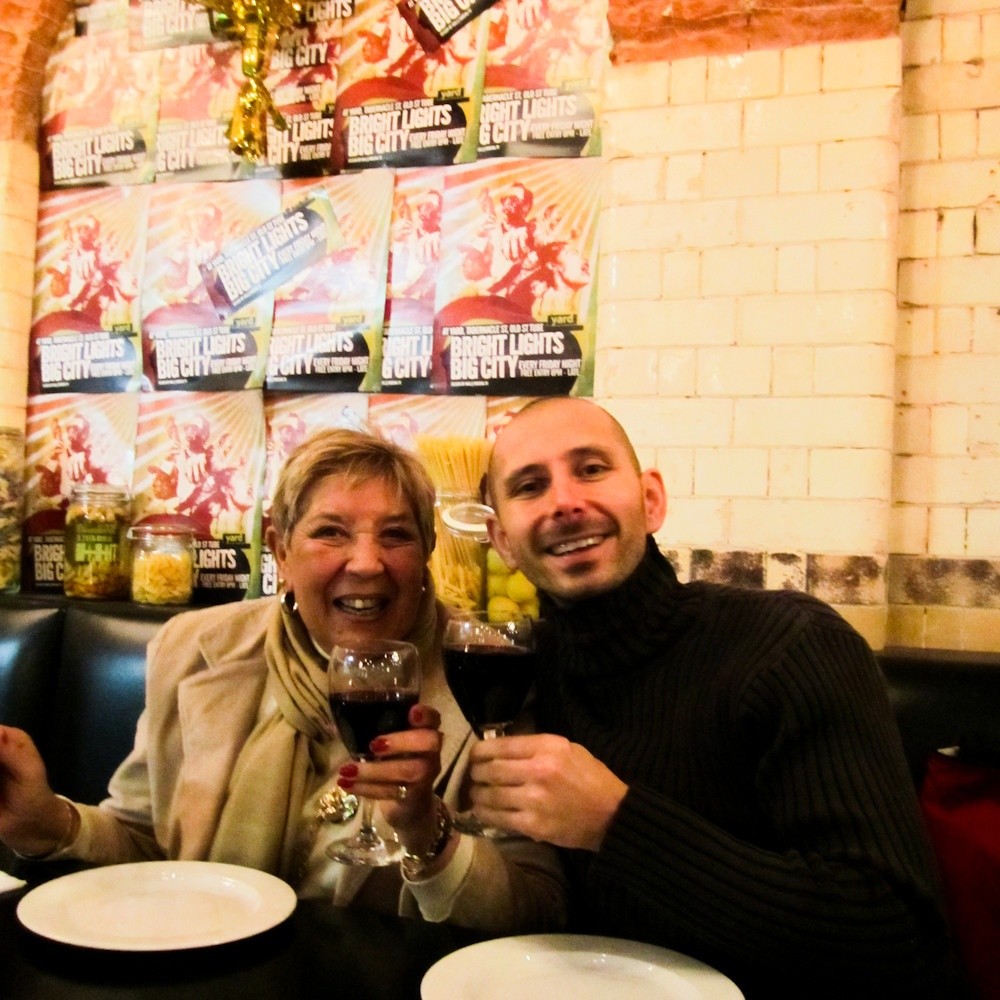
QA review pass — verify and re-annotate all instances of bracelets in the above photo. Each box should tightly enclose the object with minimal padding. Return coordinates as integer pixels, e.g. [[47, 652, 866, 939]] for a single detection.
[[54, 799, 74, 852], [402, 791, 453, 876]]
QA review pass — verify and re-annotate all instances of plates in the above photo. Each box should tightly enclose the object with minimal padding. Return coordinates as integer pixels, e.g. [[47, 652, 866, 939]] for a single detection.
[[17, 860, 298, 953], [419, 932, 749, 1000]]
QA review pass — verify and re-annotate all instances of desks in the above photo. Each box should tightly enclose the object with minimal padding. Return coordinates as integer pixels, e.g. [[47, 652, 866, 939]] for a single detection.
[[0, 878, 501, 1000]]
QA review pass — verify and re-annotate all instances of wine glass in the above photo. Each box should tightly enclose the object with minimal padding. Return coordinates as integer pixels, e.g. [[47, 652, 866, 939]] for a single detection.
[[326, 638, 424, 868], [441, 618, 538, 844]]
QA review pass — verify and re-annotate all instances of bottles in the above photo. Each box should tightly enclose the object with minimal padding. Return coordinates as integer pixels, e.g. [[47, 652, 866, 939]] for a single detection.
[[1, 425, 26, 596], [429, 493, 540, 623], [63, 486, 129, 600], [127, 525, 195, 606]]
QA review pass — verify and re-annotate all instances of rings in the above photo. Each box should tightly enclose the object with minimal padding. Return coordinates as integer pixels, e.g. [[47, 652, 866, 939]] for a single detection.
[[397, 784, 407, 799]]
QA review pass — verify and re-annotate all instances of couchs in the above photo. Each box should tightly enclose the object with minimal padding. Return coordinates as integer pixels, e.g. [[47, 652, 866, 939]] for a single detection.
[[0, 605, 1000, 805]]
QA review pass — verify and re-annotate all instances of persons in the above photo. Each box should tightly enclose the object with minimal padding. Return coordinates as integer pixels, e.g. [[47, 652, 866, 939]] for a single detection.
[[0, 429, 559, 939], [469, 394, 979, 1000]]
